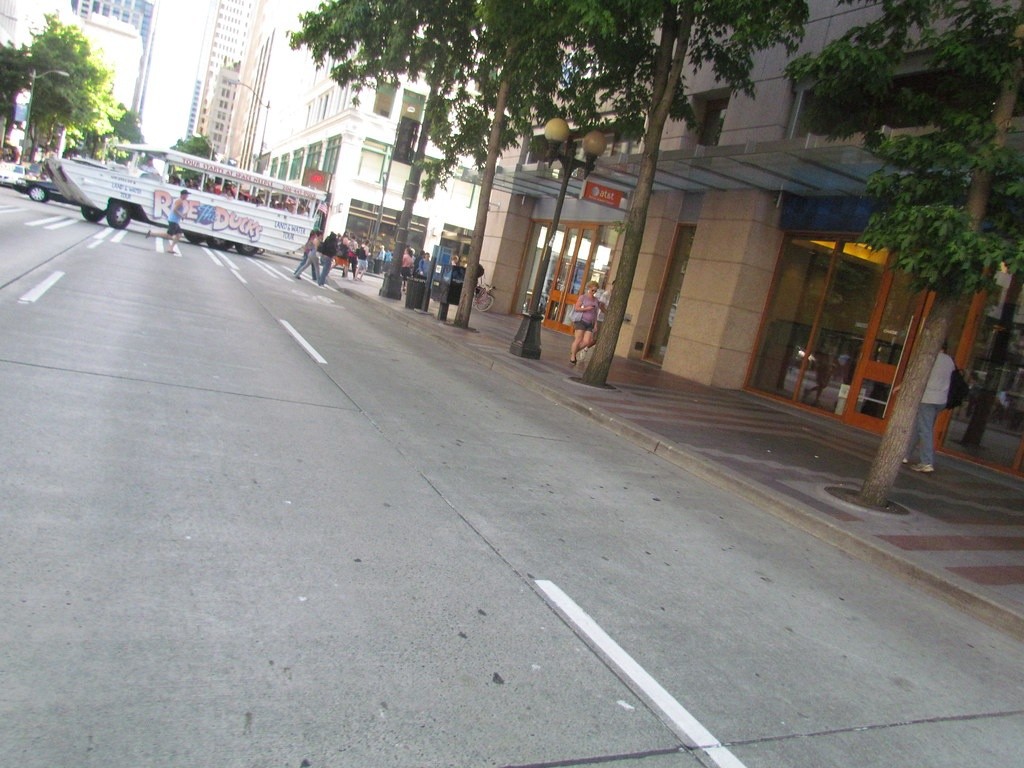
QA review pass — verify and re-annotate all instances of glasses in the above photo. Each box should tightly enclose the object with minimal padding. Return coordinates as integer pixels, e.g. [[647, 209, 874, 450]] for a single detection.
[[592, 290, 598, 292]]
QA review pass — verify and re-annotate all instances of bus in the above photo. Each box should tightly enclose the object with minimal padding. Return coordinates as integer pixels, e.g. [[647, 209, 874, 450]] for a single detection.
[[44, 144, 331, 254]]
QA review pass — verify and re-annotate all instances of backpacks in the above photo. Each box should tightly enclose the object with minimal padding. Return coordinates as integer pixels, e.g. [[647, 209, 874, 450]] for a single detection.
[[357, 245, 366, 260]]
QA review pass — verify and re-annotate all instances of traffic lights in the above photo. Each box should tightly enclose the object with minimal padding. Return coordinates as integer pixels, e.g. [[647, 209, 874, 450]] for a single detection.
[[392, 115, 420, 165]]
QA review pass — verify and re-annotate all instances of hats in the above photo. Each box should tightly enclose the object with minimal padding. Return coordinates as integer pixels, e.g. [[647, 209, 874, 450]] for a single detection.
[[313, 227, 320, 232], [181, 190, 191, 194]]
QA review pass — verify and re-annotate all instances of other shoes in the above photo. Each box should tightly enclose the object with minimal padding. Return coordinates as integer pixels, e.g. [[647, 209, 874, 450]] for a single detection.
[[911, 463, 935, 473], [569, 356, 576, 365], [146, 230, 151, 238], [903, 457, 909, 463], [167, 251, 175, 253]]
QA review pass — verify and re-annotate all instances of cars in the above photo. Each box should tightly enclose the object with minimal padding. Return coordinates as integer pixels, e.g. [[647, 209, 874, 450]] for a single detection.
[[12, 177, 78, 203], [0, 163, 31, 189]]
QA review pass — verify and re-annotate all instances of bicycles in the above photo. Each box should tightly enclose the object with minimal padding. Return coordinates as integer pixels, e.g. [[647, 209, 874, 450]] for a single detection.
[[472, 283, 497, 312]]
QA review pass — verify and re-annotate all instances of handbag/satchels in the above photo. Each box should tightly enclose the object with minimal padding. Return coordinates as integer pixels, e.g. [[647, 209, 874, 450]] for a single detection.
[[569, 304, 584, 322], [317, 241, 324, 252], [946, 356, 969, 410]]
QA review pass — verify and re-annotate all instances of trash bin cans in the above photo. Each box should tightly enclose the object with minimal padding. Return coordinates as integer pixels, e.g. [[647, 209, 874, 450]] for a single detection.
[[406, 276, 430, 312]]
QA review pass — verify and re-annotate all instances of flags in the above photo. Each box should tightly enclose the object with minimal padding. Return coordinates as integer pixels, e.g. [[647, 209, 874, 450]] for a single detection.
[[320, 201, 327, 218]]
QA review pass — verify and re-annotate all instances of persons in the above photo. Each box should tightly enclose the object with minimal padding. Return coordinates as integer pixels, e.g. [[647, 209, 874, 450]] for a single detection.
[[800, 330, 837, 407], [293, 230, 371, 287], [569, 281, 618, 364], [892, 338, 956, 472], [145, 190, 192, 254], [138, 156, 312, 218], [375, 245, 433, 292], [451, 254, 467, 269]]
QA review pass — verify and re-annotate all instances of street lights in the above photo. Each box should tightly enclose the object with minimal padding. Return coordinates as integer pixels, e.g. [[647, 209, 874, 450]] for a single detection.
[[13, 69, 70, 173], [228, 79, 271, 172], [509, 117, 609, 363]]
